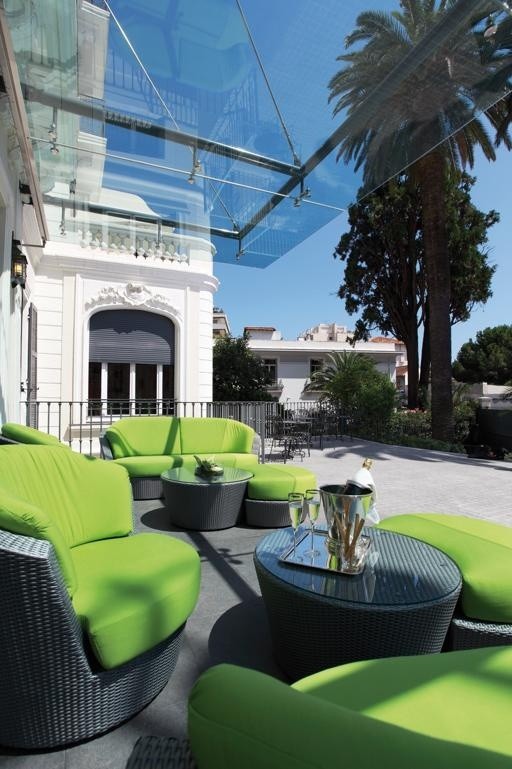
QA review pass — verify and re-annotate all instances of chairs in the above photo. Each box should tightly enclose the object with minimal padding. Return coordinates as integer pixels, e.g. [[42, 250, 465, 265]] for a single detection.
[[188, 642, 512, 769], [265, 402, 354, 464], [0, 444, 201, 750], [0, 423, 63, 445]]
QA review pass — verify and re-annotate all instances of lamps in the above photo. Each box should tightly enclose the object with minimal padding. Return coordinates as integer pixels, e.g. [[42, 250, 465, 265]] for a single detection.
[[11, 231, 28, 289]]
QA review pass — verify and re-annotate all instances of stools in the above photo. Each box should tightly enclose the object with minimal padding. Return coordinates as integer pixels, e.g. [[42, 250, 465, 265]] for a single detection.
[[371, 512, 512, 652], [244, 463, 317, 529]]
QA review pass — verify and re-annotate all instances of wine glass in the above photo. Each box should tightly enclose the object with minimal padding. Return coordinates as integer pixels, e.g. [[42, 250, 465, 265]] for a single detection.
[[303, 489, 322, 558], [287, 492, 305, 562]]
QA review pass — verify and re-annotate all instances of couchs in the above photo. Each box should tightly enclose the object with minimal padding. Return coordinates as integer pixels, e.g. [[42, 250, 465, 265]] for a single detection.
[[99, 415, 261, 501]]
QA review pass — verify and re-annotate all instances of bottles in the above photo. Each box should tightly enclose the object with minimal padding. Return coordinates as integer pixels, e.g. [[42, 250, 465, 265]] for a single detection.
[[344, 457, 374, 495]]
